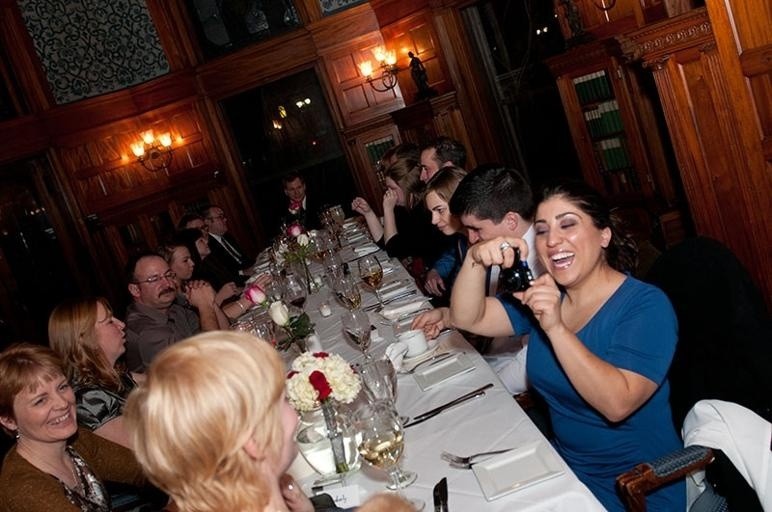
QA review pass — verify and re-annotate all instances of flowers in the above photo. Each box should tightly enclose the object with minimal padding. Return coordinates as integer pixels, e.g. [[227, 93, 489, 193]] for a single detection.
[[282, 222, 322, 295], [241, 284, 318, 354], [283, 349, 364, 476]]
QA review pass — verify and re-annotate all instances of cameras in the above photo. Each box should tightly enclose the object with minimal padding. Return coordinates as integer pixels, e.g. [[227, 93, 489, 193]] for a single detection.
[[499, 248, 535, 292]]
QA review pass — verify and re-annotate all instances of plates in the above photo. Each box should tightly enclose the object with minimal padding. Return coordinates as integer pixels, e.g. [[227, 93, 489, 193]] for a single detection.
[[402, 339, 438, 363], [470, 438, 566, 501], [414, 352, 477, 390], [376, 281, 407, 299]]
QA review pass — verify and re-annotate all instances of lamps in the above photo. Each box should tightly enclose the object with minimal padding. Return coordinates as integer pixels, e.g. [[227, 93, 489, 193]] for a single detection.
[[356, 42, 400, 100], [129, 128, 175, 174]]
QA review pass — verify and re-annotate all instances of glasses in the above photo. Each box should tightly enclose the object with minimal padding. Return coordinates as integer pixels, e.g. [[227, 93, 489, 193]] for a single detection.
[[127, 270, 178, 285]]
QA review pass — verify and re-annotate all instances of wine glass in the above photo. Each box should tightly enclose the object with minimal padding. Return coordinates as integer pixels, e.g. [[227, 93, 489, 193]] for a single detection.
[[358, 253, 388, 309], [340, 308, 377, 360], [357, 350, 426, 511], [296, 421, 362, 485]]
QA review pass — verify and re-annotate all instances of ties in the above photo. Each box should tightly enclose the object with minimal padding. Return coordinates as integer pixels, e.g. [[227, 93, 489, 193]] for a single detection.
[[221, 236, 243, 263]]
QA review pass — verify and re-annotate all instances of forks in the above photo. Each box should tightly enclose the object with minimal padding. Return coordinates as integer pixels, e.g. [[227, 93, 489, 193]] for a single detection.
[[379, 307, 432, 325], [440, 446, 516, 469], [400, 352, 449, 373]]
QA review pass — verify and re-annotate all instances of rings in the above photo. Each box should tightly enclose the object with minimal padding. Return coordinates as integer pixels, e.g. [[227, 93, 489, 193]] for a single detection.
[[499, 241, 511, 251]]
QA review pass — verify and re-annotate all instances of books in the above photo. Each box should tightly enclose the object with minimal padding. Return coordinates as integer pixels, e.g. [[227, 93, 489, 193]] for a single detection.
[[573, 70, 640, 192]]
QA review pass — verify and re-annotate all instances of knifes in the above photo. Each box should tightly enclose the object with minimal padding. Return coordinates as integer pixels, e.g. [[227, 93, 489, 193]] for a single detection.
[[413, 383, 494, 420], [403, 393, 484, 428], [365, 289, 418, 312], [433, 474, 448, 511]]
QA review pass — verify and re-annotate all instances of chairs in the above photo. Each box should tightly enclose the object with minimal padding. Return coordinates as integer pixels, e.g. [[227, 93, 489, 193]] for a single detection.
[[509, 232, 772, 512]]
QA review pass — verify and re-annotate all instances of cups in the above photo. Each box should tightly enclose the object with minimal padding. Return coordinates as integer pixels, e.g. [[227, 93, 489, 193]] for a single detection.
[[398, 329, 428, 358], [229, 203, 364, 344]]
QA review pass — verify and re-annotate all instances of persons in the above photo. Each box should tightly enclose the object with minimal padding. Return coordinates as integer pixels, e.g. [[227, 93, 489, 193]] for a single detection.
[[411, 163, 556, 444], [451, 178, 687, 512]]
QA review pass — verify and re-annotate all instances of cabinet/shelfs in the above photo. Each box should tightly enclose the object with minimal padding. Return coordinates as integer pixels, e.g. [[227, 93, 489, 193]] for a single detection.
[[541, 36, 674, 222], [355, 123, 403, 213]]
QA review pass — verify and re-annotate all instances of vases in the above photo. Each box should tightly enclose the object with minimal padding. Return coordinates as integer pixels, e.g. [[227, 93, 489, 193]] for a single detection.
[[290, 400, 364, 479], [287, 253, 322, 295], [270, 318, 322, 354]]
